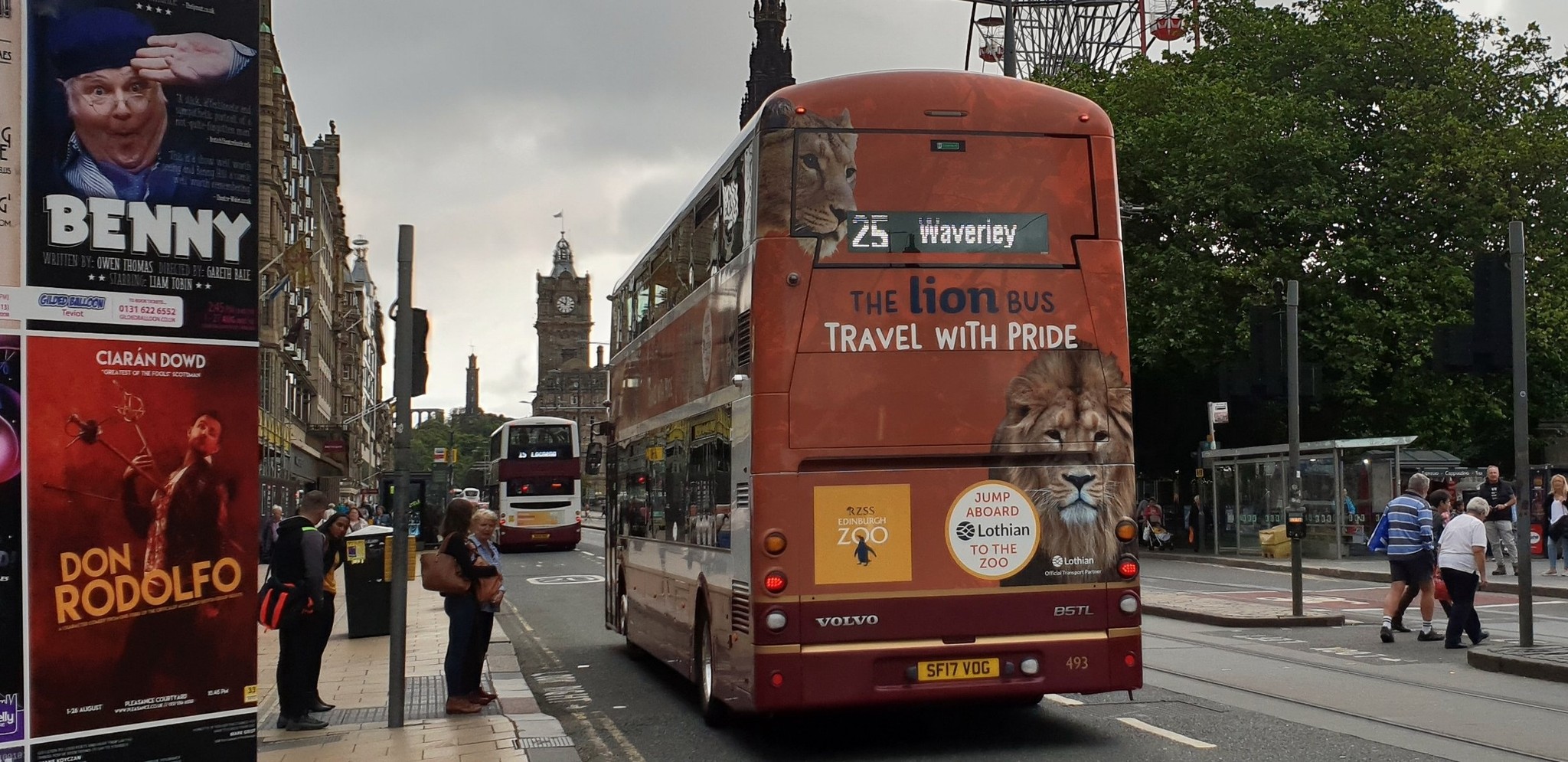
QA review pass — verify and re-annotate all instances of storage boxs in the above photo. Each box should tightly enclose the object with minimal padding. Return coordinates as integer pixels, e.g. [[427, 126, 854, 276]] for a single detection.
[[1260, 524, 1292, 557]]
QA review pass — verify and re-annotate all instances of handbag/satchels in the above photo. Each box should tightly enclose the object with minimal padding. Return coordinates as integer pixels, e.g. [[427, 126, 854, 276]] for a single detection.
[[258, 577, 313, 634], [467, 545, 504, 601], [1143, 525, 1150, 541], [1189, 526, 1193, 543], [419, 531, 473, 591], [1367, 505, 1389, 553]]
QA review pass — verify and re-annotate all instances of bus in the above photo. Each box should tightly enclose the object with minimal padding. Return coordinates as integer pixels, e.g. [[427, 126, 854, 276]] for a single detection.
[[583, 69, 1141, 732], [487, 417, 583, 553], [459, 487, 481, 505]]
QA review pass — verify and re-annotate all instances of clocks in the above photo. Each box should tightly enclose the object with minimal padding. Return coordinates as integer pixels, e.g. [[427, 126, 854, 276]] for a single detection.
[[556, 295, 575, 314]]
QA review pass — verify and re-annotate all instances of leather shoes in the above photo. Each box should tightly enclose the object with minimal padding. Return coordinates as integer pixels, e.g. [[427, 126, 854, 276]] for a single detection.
[[1470, 631, 1489, 645], [1444, 642, 1467, 649]]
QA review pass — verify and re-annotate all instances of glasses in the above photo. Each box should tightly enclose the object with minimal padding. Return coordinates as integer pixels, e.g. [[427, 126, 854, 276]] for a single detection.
[[71, 86, 155, 118]]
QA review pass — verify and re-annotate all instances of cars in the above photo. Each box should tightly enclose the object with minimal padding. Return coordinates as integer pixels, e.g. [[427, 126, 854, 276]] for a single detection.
[[649, 510, 665, 530]]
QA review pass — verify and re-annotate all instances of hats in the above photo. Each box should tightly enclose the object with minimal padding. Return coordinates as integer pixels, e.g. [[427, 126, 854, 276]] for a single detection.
[[54, 9, 163, 76]]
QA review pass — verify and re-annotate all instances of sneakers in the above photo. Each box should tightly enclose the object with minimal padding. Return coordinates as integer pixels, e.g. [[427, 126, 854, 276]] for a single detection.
[[286, 709, 329, 731], [1380, 627, 1394, 642], [276, 708, 290, 729], [1512, 563, 1520, 575], [1492, 565, 1507, 575], [1541, 569, 1558, 576], [1561, 570, 1568, 576], [1418, 625, 1445, 641], [1391, 617, 1411, 633]]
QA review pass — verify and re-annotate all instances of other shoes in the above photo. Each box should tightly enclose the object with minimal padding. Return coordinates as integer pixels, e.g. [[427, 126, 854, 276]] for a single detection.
[[309, 698, 330, 712], [446, 686, 497, 714], [1194, 548, 1198, 553], [316, 689, 336, 708]]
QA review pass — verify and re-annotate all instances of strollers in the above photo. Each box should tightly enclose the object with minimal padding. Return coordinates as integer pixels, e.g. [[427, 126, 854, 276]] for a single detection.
[[1147, 516, 1181, 553]]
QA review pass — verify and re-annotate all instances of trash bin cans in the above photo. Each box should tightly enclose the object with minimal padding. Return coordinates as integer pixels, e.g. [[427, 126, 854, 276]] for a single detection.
[[344, 525, 395, 637], [1259, 524, 1291, 559]]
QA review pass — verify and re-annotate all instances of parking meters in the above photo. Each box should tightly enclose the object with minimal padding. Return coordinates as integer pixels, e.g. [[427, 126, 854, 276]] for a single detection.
[[1286, 507, 1306, 617]]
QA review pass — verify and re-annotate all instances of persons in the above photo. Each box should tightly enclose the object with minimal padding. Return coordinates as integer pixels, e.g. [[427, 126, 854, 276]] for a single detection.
[[113, 410, 235, 724], [583, 502, 606, 520], [38, 9, 259, 211], [262, 491, 506, 730], [1134, 465, 1568, 649]]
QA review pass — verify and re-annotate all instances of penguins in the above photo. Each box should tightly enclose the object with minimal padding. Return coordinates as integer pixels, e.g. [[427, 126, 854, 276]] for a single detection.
[[854, 534, 877, 566]]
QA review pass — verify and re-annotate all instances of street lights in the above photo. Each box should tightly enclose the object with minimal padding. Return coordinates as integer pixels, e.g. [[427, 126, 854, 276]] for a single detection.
[[547, 369, 582, 454]]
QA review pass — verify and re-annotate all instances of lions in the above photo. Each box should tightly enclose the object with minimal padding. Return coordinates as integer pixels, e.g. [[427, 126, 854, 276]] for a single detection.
[[987, 338, 1136, 588]]
